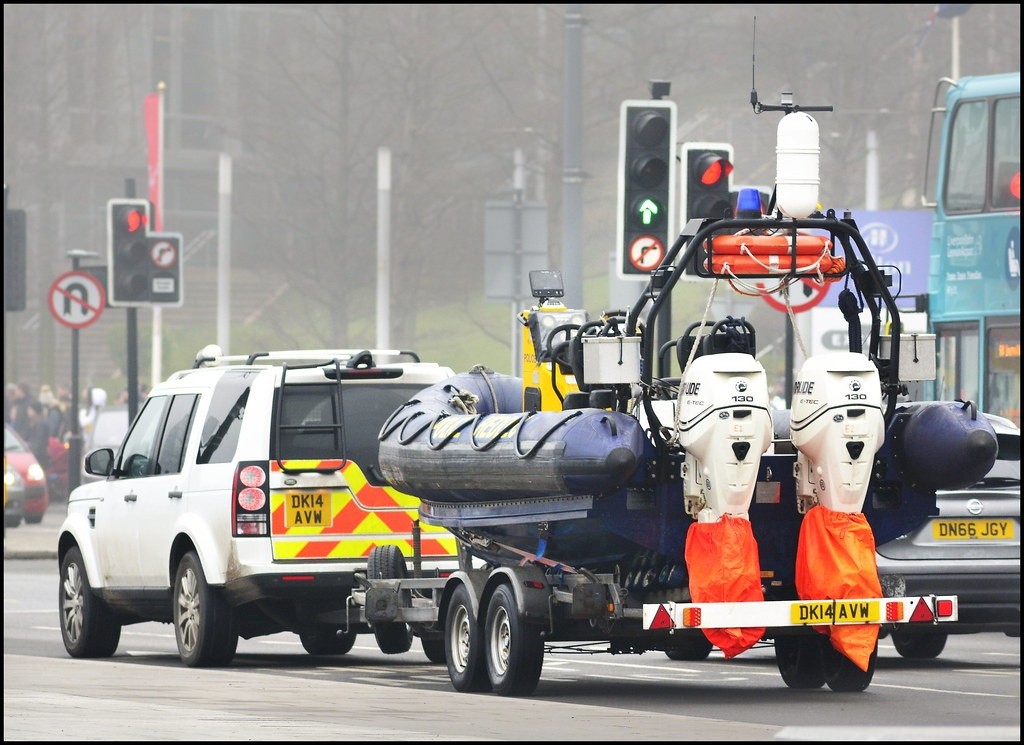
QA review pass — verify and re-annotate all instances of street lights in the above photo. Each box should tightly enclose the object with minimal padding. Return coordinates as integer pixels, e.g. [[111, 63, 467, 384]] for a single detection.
[[63, 249, 98, 503]]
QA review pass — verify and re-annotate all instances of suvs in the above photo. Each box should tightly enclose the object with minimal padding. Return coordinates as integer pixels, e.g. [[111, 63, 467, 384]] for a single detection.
[[61, 341, 462, 669]]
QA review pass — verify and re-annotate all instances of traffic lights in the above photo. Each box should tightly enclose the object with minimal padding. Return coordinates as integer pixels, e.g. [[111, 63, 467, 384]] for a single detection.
[[108, 197, 185, 307], [619, 98, 681, 285], [681, 141, 735, 282]]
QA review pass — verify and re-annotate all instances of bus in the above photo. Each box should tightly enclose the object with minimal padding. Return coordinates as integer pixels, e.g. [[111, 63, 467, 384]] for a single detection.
[[877, 70, 1019, 461]]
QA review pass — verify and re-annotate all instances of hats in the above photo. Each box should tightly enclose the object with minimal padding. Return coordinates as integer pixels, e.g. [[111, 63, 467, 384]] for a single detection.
[[29, 400, 43, 414], [37, 384, 54, 405]]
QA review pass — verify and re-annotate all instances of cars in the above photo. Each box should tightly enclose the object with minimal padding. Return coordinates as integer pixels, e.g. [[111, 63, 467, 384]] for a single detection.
[[2, 422, 49, 527], [3, 458, 25, 528], [846, 404, 1020, 658]]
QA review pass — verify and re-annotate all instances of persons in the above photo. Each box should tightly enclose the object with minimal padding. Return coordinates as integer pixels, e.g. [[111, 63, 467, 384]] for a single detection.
[[4, 382, 107, 501], [115, 382, 152, 406]]
